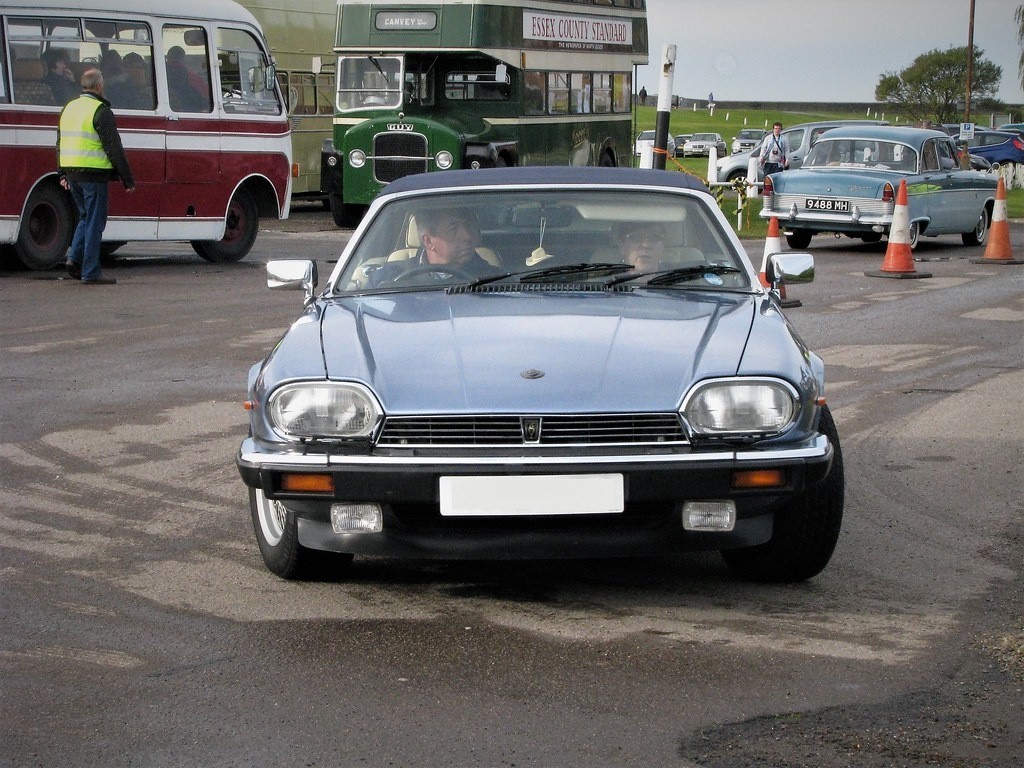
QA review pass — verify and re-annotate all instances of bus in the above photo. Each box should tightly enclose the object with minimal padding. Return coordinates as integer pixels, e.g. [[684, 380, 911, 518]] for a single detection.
[[312, 0, 649, 228], [0, 0, 301, 272], [79, 0, 398, 211]]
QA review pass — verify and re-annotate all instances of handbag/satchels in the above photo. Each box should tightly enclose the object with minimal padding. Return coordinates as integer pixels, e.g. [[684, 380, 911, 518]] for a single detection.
[[781, 156, 789, 170]]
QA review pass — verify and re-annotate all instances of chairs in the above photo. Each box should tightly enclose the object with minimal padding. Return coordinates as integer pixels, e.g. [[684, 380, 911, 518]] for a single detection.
[[591, 219, 705, 264], [12, 59, 57, 107], [388, 201, 504, 272]]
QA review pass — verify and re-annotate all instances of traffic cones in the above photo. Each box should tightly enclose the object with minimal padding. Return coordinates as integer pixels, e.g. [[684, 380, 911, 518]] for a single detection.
[[863, 178, 934, 280], [758, 213, 802, 309], [968, 175, 1024, 265]]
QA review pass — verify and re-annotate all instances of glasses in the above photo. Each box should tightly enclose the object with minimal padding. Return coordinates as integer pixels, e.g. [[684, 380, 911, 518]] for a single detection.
[[618, 232, 664, 245]]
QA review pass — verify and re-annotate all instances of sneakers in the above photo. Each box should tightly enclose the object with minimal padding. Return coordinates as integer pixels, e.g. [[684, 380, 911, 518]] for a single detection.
[[66, 259, 81, 280], [81, 272, 116, 284]]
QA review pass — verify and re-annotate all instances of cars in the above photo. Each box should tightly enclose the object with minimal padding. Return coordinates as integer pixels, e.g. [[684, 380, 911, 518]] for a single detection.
[[918, 118, 1024, 173], [756, 125, 1000, 255], [717, 120, 890, 195], [682, 132, 728, 158], [231, 163, 846, 586], [731, 128, 771, 155], [673, 133, 694, 158], [632, 129, 676, 160]]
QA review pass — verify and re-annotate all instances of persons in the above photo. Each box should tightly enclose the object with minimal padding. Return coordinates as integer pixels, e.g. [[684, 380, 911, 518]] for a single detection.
[[100, 49, 153, 111], [365, 206, 516, 287], [56, 69, 135, 283], [44, 50, 78, 103], [166, 45, 211, 113], [638, 86, 648, 106], [613, 222, 668, 281], [914, 119, 932, 129], [760, 122, 790, 181], [941, 156, 955, 168]]
